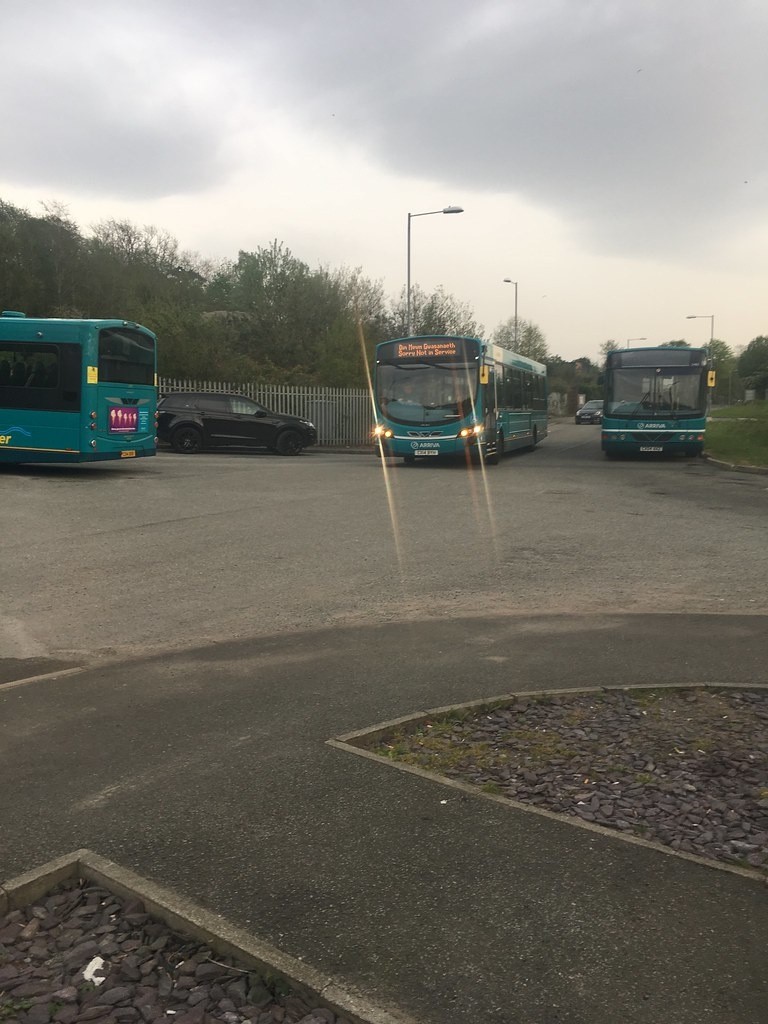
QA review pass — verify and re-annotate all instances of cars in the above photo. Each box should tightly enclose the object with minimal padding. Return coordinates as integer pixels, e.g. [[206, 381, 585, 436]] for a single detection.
[[576, 400, 604, 424], [156, 392, 318, 457]]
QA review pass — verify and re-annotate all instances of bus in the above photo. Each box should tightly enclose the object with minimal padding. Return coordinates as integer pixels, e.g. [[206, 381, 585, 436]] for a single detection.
[[603, 346, 717, 460], [0, 310, 159, 467], [370, 334, 548, 470]]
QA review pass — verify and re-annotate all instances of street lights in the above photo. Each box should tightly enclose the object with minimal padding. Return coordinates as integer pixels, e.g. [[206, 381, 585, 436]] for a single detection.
[[406, 205, 465, 335], [500, 272, 520, 353], [624, 332, 647, 348], [686, 312, 716, 349]]
